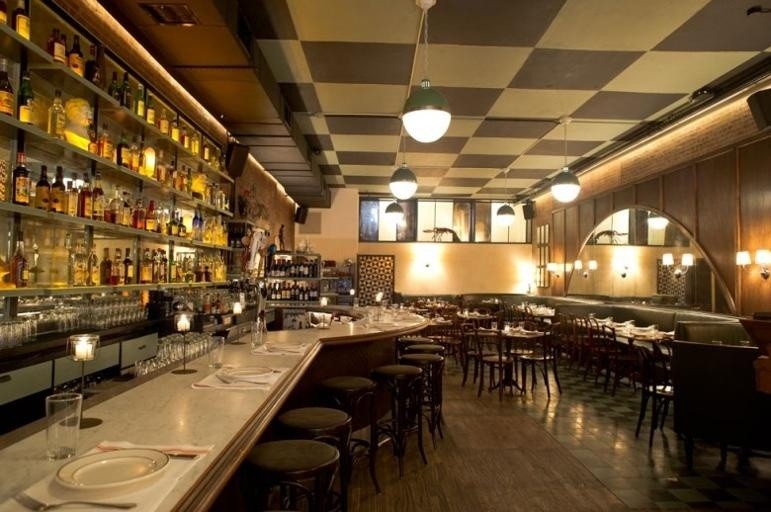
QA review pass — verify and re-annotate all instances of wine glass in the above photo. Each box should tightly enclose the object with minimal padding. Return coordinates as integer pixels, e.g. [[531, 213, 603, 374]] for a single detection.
[[136, 332, 215, 378], [54, 296, 143, 330], [0, 314, 38, 349], [519, 322, 525, 331], [490, 321, 497, 330]]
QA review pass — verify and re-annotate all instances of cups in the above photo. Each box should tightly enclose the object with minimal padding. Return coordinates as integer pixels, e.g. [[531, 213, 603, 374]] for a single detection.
[[43, 391, 84, 461], [208, 335, 226, 367], [249, 320, 265, 348]]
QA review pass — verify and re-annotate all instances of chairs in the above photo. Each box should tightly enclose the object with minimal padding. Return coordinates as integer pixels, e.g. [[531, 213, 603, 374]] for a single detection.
[[668, 339, 771, 473]]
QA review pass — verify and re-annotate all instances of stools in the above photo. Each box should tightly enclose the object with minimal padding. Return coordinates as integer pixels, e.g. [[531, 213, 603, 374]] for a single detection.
[[279, 407, 353, 505], [246, 441, 340, 511], [323, 375, 379, 495]]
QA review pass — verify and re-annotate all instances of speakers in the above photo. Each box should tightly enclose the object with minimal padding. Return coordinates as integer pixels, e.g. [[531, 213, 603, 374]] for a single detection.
[[523, 201, 533, 220], [295, 206, 307, 224], [747, 89, 771, 131], [225, 142, 250, 177]]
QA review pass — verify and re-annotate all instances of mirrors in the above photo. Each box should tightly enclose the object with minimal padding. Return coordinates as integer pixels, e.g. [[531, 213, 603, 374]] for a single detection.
[[566, 204, 736, 314]]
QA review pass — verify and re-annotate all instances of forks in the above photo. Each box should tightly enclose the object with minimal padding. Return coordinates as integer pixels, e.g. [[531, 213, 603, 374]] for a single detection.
[[5, 484, 139, 511], [215, 370, 270, 388]]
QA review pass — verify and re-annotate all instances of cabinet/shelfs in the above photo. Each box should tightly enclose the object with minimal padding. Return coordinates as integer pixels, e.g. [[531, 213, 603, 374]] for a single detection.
[[269, 256, 356, 306], [2, 1, 234, 292], [226, 219, 256, 280]]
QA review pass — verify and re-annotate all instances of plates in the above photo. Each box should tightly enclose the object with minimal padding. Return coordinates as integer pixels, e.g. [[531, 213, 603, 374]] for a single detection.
[[53, 445, 170, 491], [264, 338, 304, 350], [223, 364, 274, 378]]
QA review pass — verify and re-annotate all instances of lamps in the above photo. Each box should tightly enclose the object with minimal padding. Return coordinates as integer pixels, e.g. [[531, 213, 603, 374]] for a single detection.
[[547, 260, 561, 278], [572, 258, 599, 280], [734, 249, 769, 278], [662, 253, 694, 278]]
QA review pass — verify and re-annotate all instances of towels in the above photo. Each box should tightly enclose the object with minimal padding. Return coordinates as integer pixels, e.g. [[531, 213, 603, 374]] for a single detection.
[[0, 439, 213, 512], [191, 363, 291, 392], [252, 341, 311, 356]]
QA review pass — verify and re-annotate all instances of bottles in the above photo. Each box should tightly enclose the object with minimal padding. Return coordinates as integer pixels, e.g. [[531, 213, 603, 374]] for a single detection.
[[155, 99, 241, 186], [264, 249, 324, 305], [0, 130, 159, 293], [157, 188, 249, 286], [173, 287, 237, 313], [0, 1, 158, 186]]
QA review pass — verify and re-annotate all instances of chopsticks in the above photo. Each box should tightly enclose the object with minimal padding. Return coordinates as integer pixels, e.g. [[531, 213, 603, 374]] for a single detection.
[[98, 442, 212, 456]]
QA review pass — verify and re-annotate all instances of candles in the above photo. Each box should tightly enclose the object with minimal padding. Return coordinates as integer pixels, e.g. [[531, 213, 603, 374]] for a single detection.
[[177, 315, 190, 331], [75, 337, 92, 358]]
